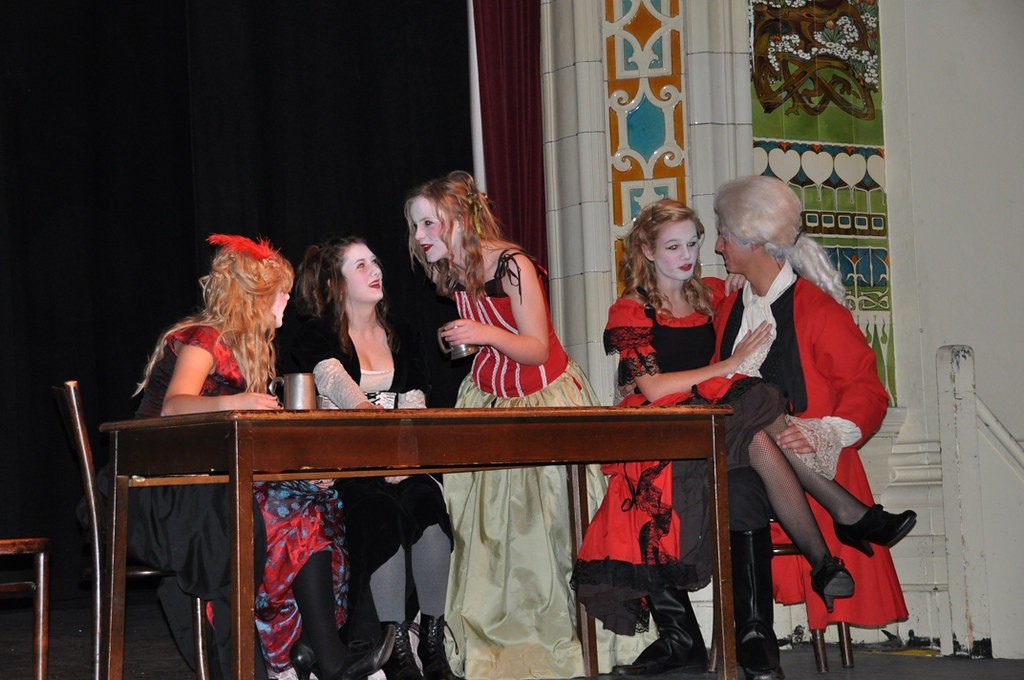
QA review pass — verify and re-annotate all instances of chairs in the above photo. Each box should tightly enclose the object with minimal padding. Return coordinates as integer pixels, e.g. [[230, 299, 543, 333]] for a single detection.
[[63, 374, 210, 680]]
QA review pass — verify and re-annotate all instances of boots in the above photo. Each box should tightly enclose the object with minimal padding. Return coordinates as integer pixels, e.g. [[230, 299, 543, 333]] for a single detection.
[[416, 611, 457, 680], [611, 584, 710, 680], [731, 523, 786, 680], [380, 618, 423, 680]]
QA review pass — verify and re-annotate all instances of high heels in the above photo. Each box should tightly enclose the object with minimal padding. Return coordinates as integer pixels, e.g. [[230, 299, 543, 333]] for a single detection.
[[288, 623, 396, 680], [810, 558, 855, 611], [833, 502, 917, 558]]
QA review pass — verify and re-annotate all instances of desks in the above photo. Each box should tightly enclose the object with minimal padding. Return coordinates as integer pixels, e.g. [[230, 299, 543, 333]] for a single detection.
[[99, 406, 736, 680]]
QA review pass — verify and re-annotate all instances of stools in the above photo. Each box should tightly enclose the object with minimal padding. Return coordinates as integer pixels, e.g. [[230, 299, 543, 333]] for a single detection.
[[702, 509, 852, 674], [0, 533, 53, 680]]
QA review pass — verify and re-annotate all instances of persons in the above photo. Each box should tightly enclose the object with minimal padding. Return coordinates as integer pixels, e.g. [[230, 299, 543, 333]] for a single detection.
[[98, 232, 454, 680], [403, 171, 659, 680], [568, 176, 917, 680]]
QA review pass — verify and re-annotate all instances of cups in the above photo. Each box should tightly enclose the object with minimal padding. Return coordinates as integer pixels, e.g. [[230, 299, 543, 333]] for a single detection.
[[268, 372, 317, 410], [438, 324, 480, 360]]
